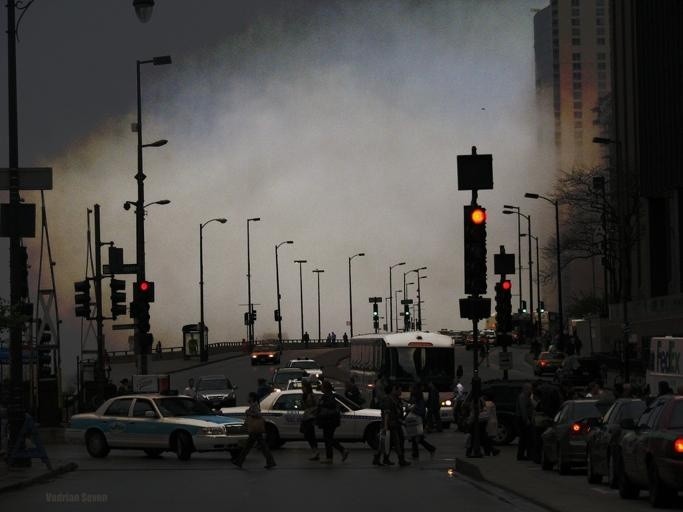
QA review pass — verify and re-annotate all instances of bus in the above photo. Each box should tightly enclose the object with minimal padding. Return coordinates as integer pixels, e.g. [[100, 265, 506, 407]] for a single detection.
[[348, 332, 463, 430]]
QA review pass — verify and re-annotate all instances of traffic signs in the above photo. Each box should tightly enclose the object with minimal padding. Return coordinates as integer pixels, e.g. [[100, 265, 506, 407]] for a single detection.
[[102, 264, 135, 275]]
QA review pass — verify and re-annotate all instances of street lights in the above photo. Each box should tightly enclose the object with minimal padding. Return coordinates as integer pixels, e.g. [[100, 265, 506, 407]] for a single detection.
[[501, 192, 564, 353], [590, 135, 632, 381], [569, 319, 580, 355], [2, 0, 155, 471], [122, 53, 175, 373], [245, 217, 428, 344], [198, 218, 228, 357]]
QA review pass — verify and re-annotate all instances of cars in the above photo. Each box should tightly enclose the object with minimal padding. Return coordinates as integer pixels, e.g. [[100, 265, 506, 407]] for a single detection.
[[62, 393, 250, 459], [453, 335, 683, 507], [218, 390, 424, 448], [193, 374, 237, 408], [439, 329, 497, 350], [250, 344, 280, 366], [268, 356, 328, 390]]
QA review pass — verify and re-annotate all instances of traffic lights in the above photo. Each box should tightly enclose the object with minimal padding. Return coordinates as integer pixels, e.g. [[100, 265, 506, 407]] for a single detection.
[[466, 205, 486, 294], [136, 303, 149, 334], [134, 333, 149, 354], [112, 279, 126, 318], [493, 282, 499, 313], [73, 279, 90, 317], [35, 316, 52, 377], [458, 296, 471, 319], [131, 279, 155, 302]]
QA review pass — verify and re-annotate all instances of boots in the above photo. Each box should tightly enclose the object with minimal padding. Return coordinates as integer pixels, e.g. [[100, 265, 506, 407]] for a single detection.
[[382, 454, 395, 465], [372, 454, 384, 466]]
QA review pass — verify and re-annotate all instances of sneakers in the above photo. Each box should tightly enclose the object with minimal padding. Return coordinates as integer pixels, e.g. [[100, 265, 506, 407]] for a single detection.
[[397, 459, 412, 466], [263, 461, 276, 468], [310, 450, 320, 460], [320, 458, 333, 464], [408, 447, 438, 459], [465, 448, 541, 464], [341, 448, 350, 462], [231, 461, 243, 468]]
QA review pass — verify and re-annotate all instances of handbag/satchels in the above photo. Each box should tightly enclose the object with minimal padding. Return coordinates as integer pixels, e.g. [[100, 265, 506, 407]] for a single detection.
[[246, 415, 267, 433]]
